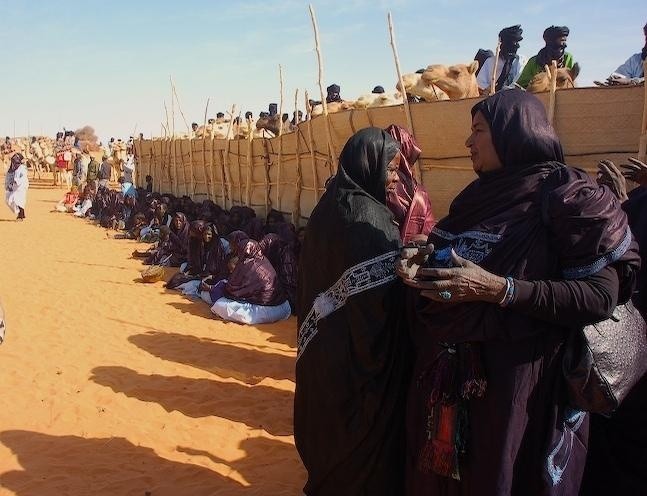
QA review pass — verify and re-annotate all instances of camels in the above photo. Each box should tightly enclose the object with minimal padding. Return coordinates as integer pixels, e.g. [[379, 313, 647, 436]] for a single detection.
[[396, 68, 451, 103], [193, 100, 350, 140], [352, 88, 403, 108], [527, 60, 580, 95], [422, 62, 479, 99]]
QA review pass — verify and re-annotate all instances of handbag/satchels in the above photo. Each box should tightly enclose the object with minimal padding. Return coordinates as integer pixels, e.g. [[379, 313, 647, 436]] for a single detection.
[[561, 297, 647, 411]]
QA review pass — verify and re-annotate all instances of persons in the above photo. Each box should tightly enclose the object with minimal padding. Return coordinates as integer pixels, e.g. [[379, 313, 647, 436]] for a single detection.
[[594, 23, 647, 86], [5, 152, 28, 222], [295, 88, 646, 496], [192, 25, 580, 138], [29, 130, 305, 326], [1, 136, 12, 154]]
